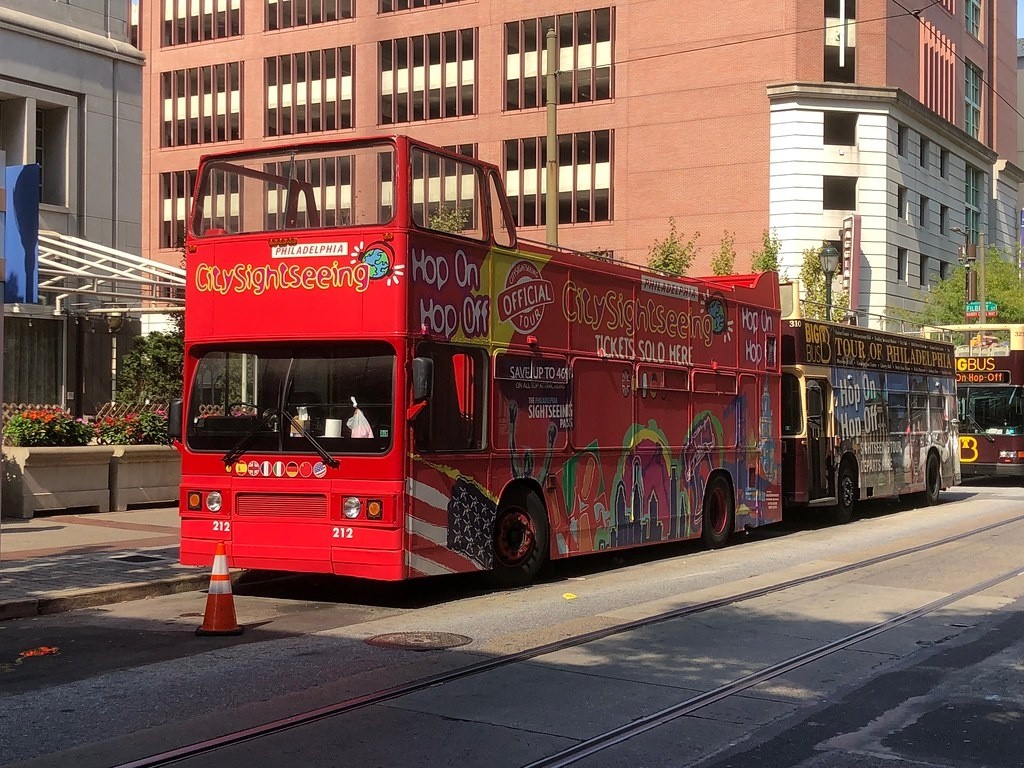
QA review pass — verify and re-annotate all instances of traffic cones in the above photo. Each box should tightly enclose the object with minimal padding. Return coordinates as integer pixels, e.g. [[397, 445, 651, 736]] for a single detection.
[[194, 538, 245, 636]]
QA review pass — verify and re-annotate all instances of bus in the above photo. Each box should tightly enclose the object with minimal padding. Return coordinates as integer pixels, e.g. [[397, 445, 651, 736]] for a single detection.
[[777, 279, 961, 525], [918, 323, 1024, 484], [167, 135, 781, 590]]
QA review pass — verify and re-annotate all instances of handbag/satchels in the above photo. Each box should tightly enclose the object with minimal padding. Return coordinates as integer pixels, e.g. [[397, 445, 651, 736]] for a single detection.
[[347, 396, 374, 438]]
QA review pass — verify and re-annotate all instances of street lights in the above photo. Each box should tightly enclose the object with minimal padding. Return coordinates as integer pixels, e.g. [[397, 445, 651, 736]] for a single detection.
[[948, 226, 971, 346], [817, 243, 839, 321]]
